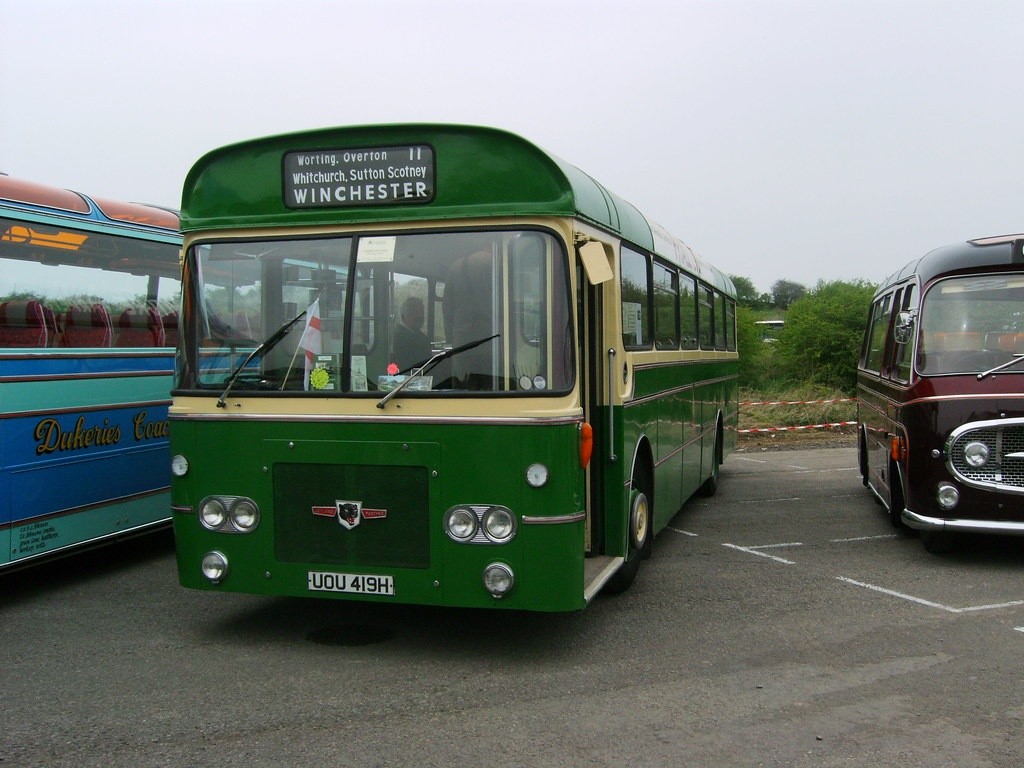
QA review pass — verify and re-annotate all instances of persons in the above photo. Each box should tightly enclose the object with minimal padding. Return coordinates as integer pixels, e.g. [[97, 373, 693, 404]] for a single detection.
[[442, 238, 515, 390], [391, 296, 433, 373]]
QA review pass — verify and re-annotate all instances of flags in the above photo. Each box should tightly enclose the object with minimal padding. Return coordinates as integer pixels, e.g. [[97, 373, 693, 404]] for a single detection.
[[298, 299, 322, 391]]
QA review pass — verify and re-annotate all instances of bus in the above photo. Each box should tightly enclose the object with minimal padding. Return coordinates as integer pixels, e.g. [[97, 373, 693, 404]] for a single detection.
[[165, 122, 741, 613], [1, 175, 192, 578], [856, 234, 1024, 555]]
